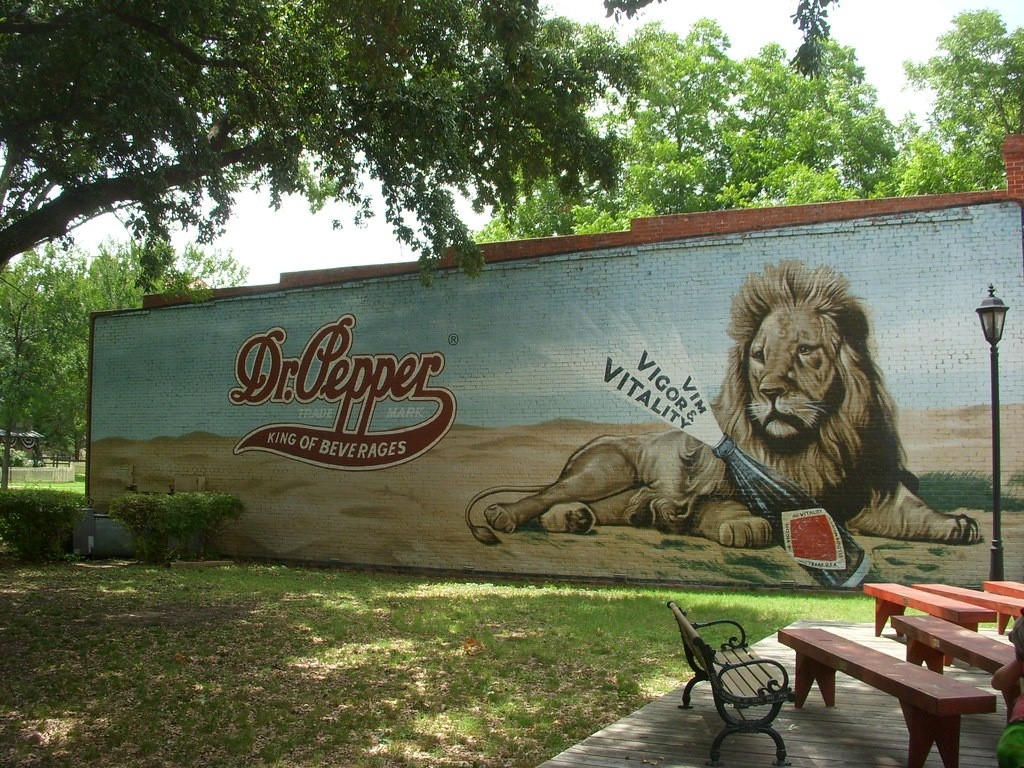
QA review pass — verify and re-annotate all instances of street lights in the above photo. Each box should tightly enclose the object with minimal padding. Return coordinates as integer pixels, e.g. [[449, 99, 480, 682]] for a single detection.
[[975, 280, 1010, 583]]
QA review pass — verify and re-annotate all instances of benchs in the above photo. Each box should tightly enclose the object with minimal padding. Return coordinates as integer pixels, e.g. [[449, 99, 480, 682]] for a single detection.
[[912, 584, 1024, 623], [890, 615, 1016, 722], [778, 629, 996, 768], [667, 598, 795, 768], [983, 581, 1024, 597], [863, 583, 997, 637]]
